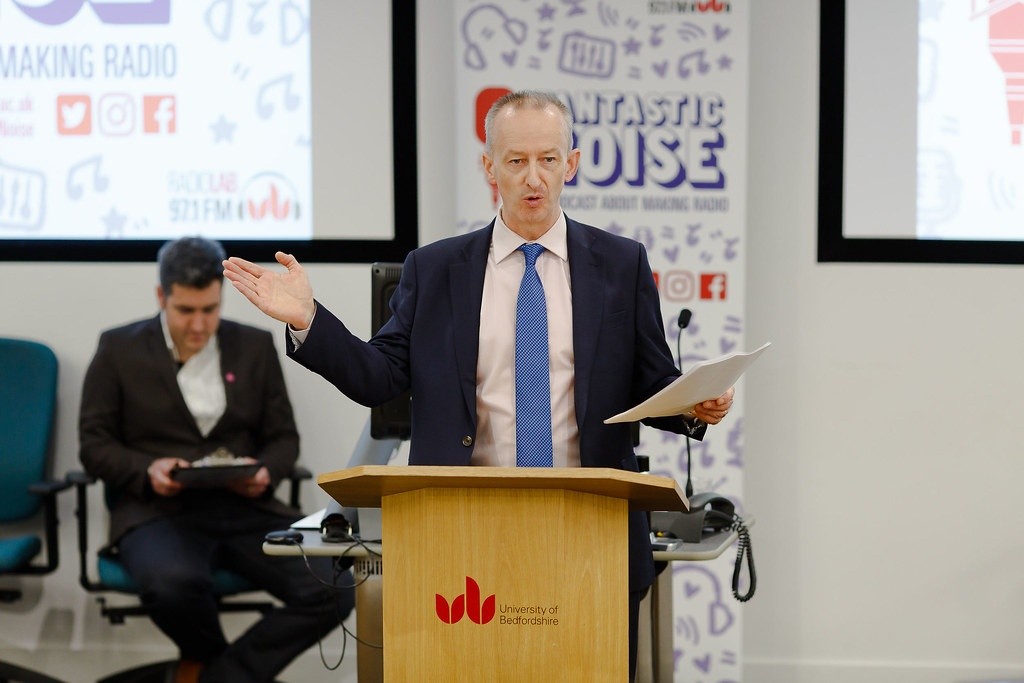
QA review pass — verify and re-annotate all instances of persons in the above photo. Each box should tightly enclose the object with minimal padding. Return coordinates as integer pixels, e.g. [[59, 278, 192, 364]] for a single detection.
[[78, 237, 357, 683], [222, 91, 735, 683]]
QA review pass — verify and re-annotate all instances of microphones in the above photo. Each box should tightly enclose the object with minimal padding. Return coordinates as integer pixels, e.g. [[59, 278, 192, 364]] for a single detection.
[[678, 309, 692, 498]]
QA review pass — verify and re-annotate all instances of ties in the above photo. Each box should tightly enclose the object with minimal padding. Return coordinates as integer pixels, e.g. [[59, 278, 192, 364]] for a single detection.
[[516, 244, 553, 467]]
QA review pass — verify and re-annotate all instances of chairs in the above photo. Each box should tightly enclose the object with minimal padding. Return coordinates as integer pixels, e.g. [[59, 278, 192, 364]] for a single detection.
[[65, 465, 328, 683], [0, 337, 73, 683]]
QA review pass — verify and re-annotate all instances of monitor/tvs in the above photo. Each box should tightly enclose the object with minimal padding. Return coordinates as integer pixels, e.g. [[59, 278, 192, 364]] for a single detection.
[[371, 263, 413, 443]]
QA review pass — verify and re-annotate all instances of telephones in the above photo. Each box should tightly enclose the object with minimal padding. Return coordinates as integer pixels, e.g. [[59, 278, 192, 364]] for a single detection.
[[648, 491, 736, 544]]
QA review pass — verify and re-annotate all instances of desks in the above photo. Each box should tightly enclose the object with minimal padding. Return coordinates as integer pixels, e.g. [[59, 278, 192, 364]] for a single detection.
[[262, 505, 758, 683]]
